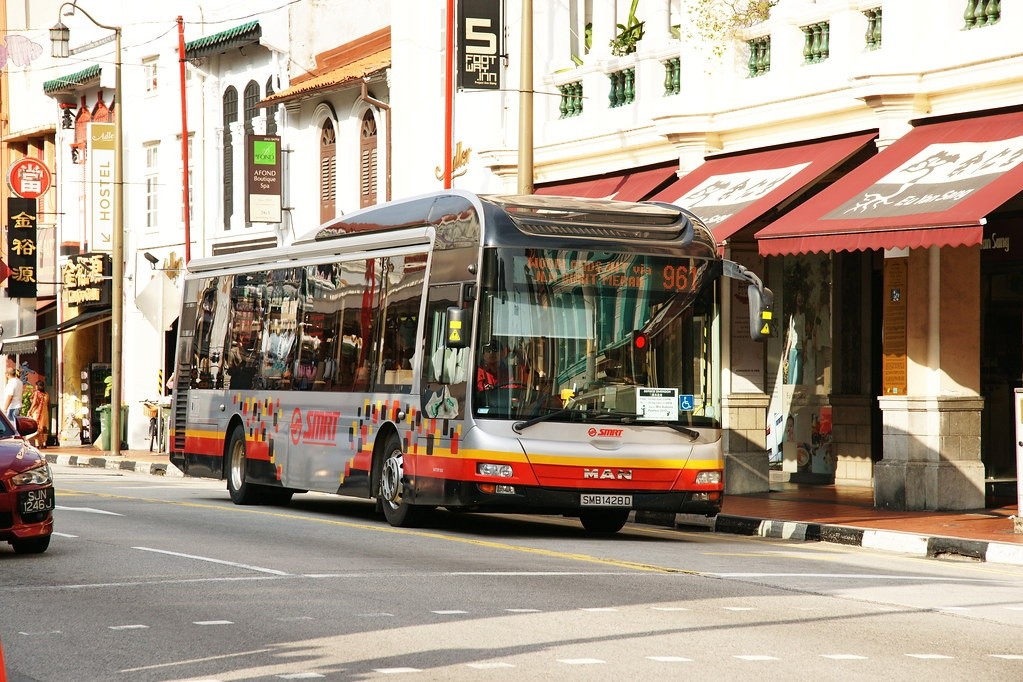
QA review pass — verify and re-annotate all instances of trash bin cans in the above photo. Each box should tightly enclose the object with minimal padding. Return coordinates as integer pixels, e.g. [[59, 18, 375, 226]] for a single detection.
[[95, 403, 127, 451]]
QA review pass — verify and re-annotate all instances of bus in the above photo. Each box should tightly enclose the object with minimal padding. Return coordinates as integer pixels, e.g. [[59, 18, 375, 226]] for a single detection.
[[164, 190, 777, 539]]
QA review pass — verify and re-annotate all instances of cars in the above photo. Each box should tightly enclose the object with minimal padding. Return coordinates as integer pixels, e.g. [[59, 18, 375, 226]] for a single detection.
[[0, 405, 57, 554]]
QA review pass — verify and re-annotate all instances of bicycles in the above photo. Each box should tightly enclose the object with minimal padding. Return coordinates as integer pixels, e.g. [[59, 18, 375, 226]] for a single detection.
[[138, 398, 165, 453]]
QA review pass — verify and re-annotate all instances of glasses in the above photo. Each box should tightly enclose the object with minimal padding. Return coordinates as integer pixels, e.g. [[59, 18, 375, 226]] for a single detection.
[[483, 348, 501, 353]]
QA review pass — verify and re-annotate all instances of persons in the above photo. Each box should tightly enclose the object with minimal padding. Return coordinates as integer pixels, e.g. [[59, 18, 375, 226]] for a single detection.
[[802, 260, 831, 384], [477, 340, 536, 391], [27, 380, 50, 448], [3, 367, 23, 428], [783, 291, 808, 384], [786, 416, 796, 442]]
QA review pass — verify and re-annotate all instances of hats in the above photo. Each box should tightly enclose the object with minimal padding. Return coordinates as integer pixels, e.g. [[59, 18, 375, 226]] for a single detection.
[[481, 340, 503, 350]]
